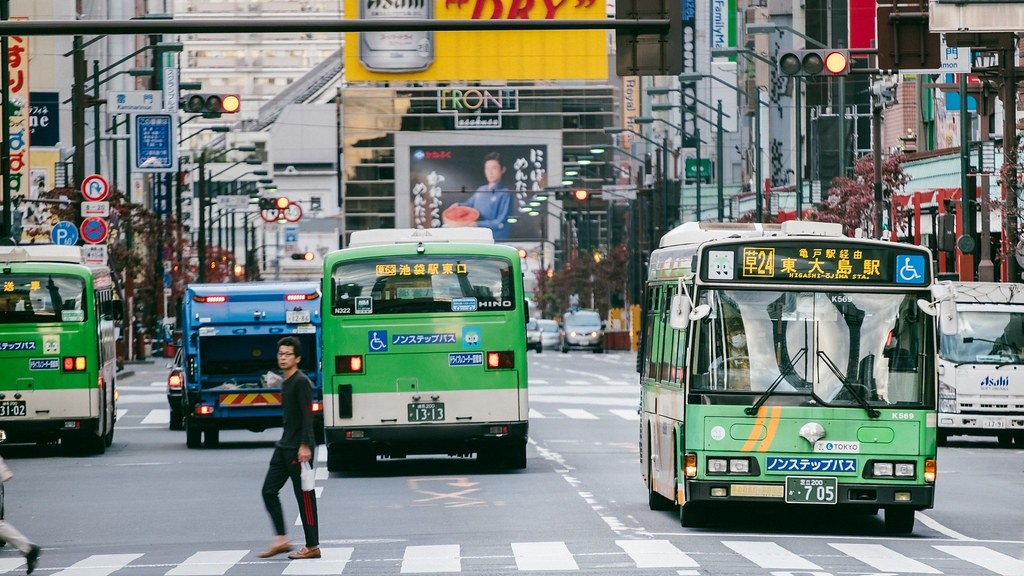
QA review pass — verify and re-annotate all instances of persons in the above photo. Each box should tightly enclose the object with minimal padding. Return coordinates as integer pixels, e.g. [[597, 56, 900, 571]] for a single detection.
[[706, 317, 752, 371], [453, 151, 513, 239], [0, 455, 41, 576], [257, 335, 321, 558]]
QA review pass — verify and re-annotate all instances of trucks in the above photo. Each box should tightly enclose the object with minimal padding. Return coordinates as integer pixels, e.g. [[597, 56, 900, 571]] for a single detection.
[[876, 282, 1024, 447]]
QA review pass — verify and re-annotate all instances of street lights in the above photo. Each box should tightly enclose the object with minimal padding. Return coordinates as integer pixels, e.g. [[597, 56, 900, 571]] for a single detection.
[[646, 87, 730, 225], [710, 46, 811, 220], [69, 13, 173, 246], [743, 22, 855, 220], [677, 71, 772, 223], [523, 101, 707, 310], [69, 38, 278, 339]]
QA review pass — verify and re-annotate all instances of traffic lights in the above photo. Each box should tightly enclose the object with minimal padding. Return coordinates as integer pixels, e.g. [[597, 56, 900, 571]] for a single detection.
[[776, 47, 852, 79], [258, 197, 290, 210], [290, 251, 316, 262], [182, 93, 241, 117], [554, 190, 590, 200]]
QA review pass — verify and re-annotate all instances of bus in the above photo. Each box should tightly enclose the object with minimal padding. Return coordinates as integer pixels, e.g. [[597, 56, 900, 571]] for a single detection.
[[635, 220, 958, 537], [183, 281, 321, 448], [315, 229, 534, 474], [1, 246, 125, 452]]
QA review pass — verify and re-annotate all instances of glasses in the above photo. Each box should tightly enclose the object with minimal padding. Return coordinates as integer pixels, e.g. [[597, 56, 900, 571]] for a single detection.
[[276, 351, 295, 356]]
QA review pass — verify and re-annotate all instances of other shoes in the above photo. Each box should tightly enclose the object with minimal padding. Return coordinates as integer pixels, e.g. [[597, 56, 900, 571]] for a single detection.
[[24, 544, 41, 575]]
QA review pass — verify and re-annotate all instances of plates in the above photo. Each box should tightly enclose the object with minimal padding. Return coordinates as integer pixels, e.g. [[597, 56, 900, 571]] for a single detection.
[[442, 206, 480, 227]]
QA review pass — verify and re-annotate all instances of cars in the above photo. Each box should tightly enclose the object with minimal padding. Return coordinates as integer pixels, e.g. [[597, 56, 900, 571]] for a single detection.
[[524, 322, 544, 352], [538, 319, 563, 349], [560, 310, 606, 354], [166, 344, 186, 431]]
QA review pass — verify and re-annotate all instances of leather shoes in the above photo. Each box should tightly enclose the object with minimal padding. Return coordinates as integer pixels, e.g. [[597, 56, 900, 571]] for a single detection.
[[257, 541, 292, 559], [288, 546, 321, 559]]
